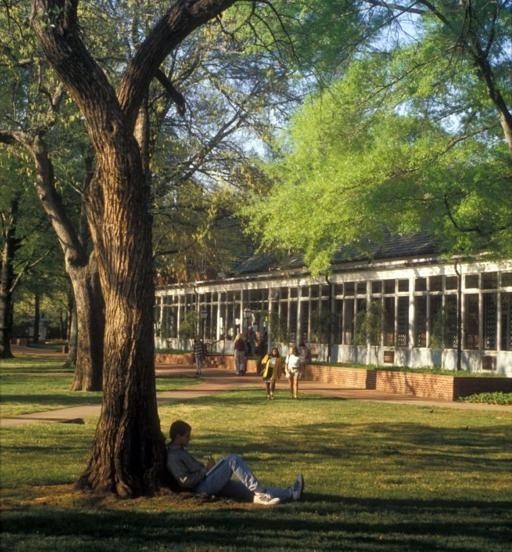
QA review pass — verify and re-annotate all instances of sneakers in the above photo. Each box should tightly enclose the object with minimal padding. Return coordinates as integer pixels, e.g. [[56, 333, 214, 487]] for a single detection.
[[253, 495, 280, 505], [289, 474, 304, 501]]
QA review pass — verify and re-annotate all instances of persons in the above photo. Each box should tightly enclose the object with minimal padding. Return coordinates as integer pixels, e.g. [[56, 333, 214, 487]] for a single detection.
[[192, 335, 207, 378], [232, 322, 310, 399], [166, 419, 304, 505]]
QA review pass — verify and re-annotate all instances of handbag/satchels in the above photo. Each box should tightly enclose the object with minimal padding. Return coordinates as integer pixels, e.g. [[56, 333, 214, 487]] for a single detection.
[[257, 364, 266, 375], [176, 472, 206, 488]]
